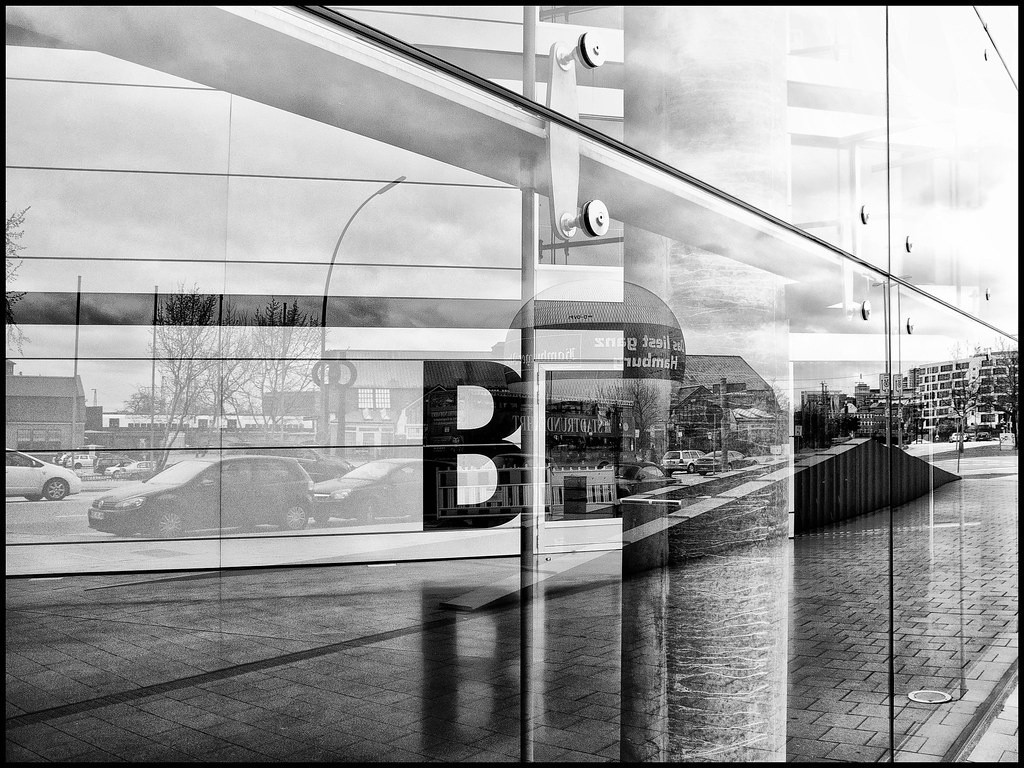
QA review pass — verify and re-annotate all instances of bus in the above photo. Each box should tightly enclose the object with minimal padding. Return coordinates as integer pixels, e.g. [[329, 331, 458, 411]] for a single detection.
[[545, 401, 637, 469]]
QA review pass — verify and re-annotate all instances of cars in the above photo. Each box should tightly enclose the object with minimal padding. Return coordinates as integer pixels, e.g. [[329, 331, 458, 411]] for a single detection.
[[697, 450, 744, 476], [743, 449, 778, 466], [311, 458, 470, 526], [223, 442, 354, 480], [550, 445, 613, 476], [87, 452, 318, 538], [52, 450, 157, 479], [476, 454, 562, 512], [5, 448, 81, 500], [581, 461, 682, 505], [661, 449, 706, 473], [949, 433, 991, 443]]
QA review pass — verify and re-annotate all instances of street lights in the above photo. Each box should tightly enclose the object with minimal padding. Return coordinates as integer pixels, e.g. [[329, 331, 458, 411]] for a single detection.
[[322, 176, 408, 457]]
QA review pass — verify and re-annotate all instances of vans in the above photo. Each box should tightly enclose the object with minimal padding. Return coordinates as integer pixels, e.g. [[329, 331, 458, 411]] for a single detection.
[[163, 433, 256, 469]]
